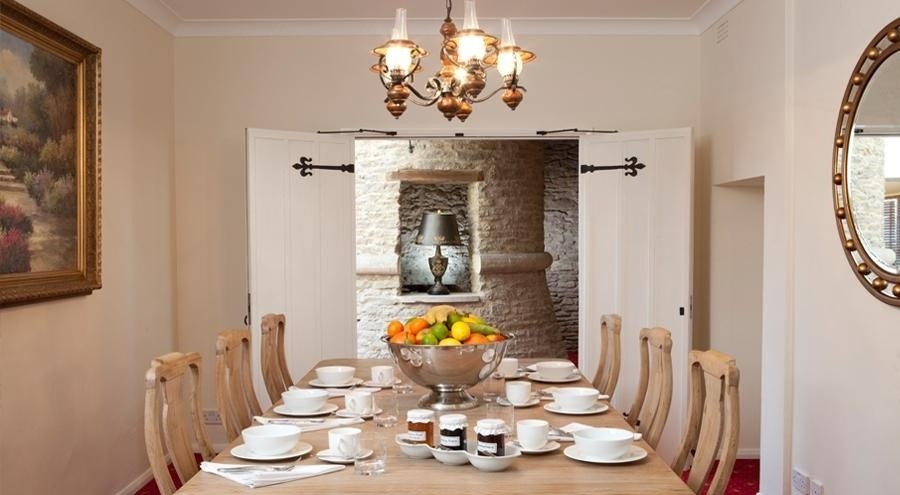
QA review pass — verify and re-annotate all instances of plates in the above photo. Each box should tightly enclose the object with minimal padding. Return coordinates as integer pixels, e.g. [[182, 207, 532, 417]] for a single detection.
[[391, 434, 522, 472], [493, 364, 650, 464], [229, 378, 401, 464]]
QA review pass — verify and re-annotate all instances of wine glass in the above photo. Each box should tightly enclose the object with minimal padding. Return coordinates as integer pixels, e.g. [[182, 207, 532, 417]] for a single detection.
[[380, 332, 516, 414]]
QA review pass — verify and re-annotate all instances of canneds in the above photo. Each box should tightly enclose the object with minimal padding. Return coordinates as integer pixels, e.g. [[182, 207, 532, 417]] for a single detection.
[[476, 419, 505, 456], [407, 409, 434, 447], [439, 414, 467, 451]]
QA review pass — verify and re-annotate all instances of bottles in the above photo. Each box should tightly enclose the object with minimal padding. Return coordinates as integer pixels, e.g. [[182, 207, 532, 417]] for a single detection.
[[402, 409, 508, 459]]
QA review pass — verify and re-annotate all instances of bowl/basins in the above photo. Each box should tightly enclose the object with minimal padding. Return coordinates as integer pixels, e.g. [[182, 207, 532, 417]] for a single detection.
[[316, 364, 359, 383]]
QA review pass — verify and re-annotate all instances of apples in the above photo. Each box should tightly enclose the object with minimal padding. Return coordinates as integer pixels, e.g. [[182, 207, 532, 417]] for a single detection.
[[387, 314, 504, 346]]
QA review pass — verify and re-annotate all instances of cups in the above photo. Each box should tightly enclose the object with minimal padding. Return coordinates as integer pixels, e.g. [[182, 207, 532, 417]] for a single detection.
[[372, 365, 394, 385], [392, 359, 414, 396], [352, 431, 392, 476], [498, 358, 519, 377]]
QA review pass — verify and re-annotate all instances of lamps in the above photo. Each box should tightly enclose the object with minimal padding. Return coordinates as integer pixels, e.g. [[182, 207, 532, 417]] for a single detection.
[[414, 209, 464, 296], [365, 0, 538, 124]]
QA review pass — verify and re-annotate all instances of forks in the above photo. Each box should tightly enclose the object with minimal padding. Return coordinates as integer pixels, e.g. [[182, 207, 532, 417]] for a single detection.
[[216, 454, 302, 474]]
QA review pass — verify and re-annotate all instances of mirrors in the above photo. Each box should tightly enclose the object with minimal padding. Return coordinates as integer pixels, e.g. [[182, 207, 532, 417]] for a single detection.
[[831, 16, 900, 309]]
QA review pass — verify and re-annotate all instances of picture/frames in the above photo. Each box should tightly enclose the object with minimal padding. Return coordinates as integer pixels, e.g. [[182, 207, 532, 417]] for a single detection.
[[0, 1, 105, 312]]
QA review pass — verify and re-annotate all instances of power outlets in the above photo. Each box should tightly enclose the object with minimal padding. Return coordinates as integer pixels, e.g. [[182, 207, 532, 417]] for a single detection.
[[791, 462, 825, 495]]
